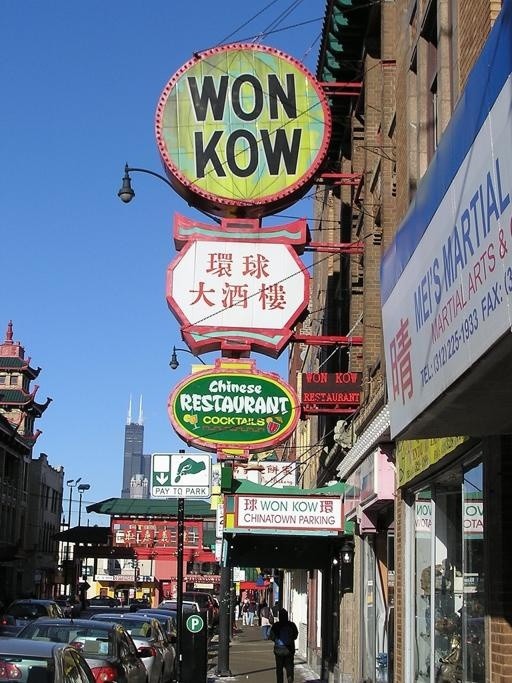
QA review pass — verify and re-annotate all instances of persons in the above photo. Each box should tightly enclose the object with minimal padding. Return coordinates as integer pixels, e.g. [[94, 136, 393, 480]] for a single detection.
[[241, 598, 298, 683]]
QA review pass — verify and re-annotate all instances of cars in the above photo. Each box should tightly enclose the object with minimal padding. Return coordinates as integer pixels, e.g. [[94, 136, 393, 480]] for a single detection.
[[0, 590, 222, 683]]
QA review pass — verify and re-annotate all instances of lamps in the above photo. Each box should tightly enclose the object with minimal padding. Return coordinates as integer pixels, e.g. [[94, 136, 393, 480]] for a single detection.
[[340, 539, 355, 563]]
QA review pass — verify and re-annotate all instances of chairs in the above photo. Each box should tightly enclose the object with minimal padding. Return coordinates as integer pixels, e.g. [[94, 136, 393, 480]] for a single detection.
[[129, 629, 150, 636], [83, 640, 105, 654]]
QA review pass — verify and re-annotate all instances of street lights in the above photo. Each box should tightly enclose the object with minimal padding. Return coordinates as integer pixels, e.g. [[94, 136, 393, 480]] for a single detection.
[[63, 477, 81, 595], [132, 549, 139, 599], [78, 483, 91, 527]]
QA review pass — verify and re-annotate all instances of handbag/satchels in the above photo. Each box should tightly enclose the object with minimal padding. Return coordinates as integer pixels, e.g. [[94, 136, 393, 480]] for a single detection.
[[262, 617, 272, 626]]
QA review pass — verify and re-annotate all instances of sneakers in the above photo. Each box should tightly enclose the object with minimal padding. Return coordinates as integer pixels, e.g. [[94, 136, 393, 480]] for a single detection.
[[243, 624, 246, 626], [250, 624, 255, 626], [247, 623, 250, 625]]
